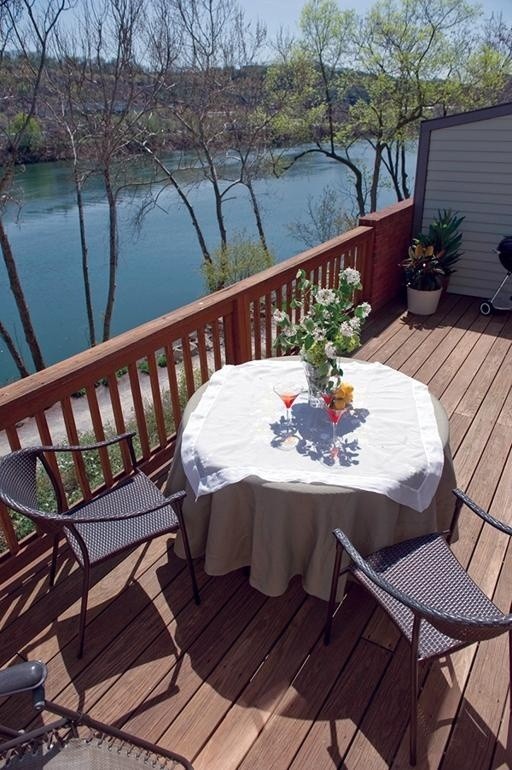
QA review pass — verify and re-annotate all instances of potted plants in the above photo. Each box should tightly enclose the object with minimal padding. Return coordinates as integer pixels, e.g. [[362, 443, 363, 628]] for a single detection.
[[398, 241, 446, 316], [410, 204, 467, 298]]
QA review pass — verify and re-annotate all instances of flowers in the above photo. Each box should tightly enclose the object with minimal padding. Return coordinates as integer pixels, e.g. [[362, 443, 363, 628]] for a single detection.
[[272, 259, 372, 377]]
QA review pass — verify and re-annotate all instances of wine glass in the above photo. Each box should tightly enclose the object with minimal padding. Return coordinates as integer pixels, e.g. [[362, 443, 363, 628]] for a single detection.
[[320, 401, 352, 461], [272, 381, 308, 449]]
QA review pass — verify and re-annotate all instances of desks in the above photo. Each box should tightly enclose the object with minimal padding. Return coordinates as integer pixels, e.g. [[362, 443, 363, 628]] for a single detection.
[[165, 356, 459, 602]]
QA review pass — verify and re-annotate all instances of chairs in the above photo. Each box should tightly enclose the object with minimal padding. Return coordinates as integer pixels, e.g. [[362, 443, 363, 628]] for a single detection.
[[0, 661, 196, 769], [0, 432, 203, 657], [323, 488, 512, 767]]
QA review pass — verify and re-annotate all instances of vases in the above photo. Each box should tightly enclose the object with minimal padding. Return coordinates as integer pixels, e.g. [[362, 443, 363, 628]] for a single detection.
[[307, 356, 331, 410]]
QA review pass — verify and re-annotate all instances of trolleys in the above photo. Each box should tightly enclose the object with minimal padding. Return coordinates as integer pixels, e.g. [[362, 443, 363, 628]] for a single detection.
[[477, 234, 512, 316]]
